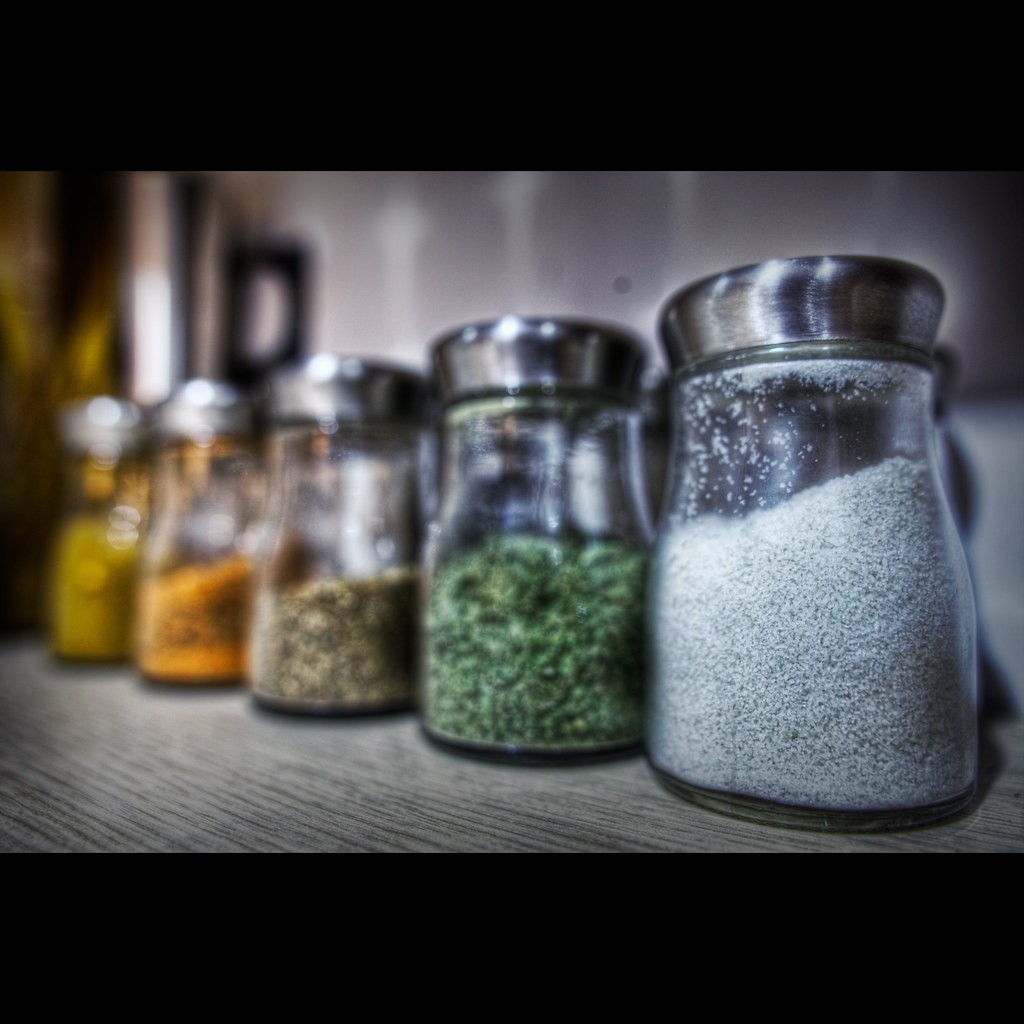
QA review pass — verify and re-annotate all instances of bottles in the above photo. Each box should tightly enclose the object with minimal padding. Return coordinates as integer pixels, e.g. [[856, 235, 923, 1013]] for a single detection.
[[243, 349, 438, 726], [634, 251, 990, 836], [44, 395, 146, 663], [412, 312, 661, 772], [128, 378, 256, 689]]
